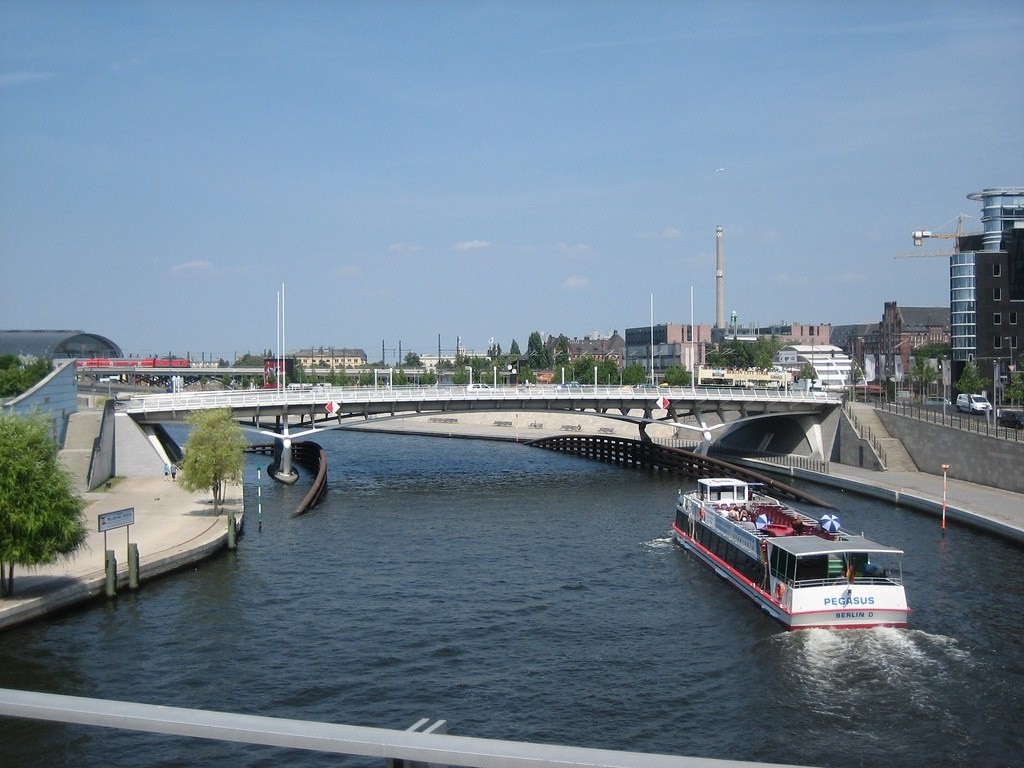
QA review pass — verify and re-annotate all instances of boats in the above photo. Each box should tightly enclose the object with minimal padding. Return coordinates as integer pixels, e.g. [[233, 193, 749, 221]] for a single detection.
[[673, 478, 913, 632]]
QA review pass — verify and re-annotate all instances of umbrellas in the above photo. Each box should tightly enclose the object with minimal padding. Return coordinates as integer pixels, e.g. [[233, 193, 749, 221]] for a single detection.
[[756, 514, 771, 530], [819, 514, 841, 532]]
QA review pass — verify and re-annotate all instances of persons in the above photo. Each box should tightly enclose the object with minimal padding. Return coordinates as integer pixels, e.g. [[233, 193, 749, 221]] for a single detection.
[[730, 507, 740, 521], [170, 462, 179, 481], [740, 508, 748, 521], [791, 514, 806, 535], [164, 463, 170, 481]]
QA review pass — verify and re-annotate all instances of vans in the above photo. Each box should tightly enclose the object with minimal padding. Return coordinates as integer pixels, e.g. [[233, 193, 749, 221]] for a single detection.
[[928, 396, 952, 405], [896, 391, 910, 405], [956, 394, 992, 415]]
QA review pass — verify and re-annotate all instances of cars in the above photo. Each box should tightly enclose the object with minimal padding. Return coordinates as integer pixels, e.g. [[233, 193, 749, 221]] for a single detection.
[[554, 382, 583, 393], [997, 409, 1024, 429]]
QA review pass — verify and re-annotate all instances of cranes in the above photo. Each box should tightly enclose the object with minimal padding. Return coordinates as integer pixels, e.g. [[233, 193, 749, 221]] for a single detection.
[[894, 212, 985, 259]]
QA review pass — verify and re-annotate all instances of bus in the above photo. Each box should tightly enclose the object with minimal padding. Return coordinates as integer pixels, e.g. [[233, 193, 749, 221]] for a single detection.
[[848, 385, 886, 404], [287, 384, 332, 397]]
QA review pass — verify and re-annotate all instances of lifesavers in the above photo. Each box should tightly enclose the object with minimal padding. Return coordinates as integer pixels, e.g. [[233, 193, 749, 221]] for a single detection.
[[699, 507, 704, 518], [775, 582, 784, 600]]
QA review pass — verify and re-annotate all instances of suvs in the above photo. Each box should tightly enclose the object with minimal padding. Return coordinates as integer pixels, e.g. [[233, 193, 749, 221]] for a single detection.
[[468, 383, 497, 393], [633, 385, 660, 393]]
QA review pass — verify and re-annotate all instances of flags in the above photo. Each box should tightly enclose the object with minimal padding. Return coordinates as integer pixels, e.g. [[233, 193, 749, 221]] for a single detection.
[[488, 337, 493, 344]]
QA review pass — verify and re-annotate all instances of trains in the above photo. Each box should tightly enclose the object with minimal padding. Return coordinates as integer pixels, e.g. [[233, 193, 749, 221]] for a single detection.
[[76, 357, 190, 368]]
